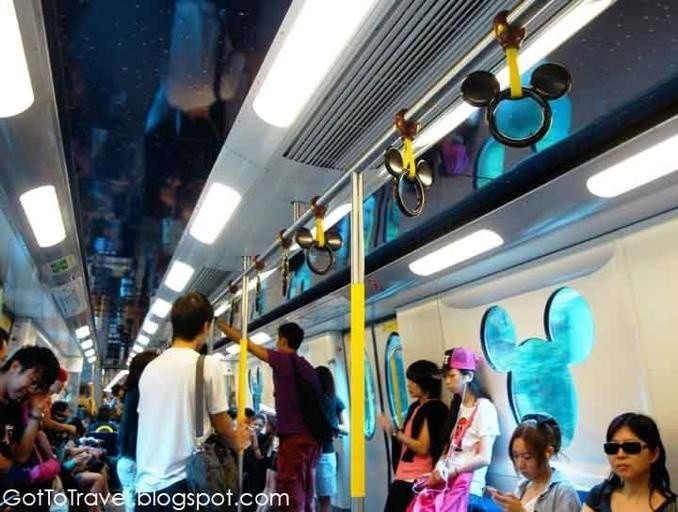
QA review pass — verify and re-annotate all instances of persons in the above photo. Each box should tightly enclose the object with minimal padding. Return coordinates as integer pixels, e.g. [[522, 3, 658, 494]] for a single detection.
[[0, 292, 350, 512], [489, 413, 582, 512], [131, 11, 252, 218], [581, 412, 678, 512], [376, 359, 448, 512], [404, 347, 501, 512]]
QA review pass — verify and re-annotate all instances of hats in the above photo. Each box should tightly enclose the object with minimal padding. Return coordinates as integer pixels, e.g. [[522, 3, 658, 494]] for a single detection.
[[57, 367, 66, 381], [430, 348, 478, 375]]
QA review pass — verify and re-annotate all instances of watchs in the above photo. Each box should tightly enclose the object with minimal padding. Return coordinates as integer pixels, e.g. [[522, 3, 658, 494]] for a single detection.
[[391, 428, 398, 438]]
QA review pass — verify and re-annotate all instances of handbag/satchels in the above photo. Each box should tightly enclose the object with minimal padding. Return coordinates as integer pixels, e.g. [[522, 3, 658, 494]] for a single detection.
[[32, 459, 60, 480], [187, 428, 240, 511], [49, 489, 70, 512], [297, 377, 334, 441], [412, 472, 472, 511], [256, 469, 318, 512]]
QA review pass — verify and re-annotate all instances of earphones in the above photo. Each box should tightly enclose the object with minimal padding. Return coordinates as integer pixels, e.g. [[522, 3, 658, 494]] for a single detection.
[[463, 375, 470, 383]]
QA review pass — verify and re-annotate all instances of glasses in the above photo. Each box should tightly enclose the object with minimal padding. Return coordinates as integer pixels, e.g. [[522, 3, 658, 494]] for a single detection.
[[603, 441, 649, 455]]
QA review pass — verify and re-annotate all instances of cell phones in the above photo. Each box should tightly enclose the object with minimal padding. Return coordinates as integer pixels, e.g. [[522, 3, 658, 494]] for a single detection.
[[487, 486, 502, 496], [416, 478, 427, 489]]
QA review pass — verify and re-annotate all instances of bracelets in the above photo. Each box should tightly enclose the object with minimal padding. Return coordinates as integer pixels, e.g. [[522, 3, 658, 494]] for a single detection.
[[454, 467, 460, 476]]
